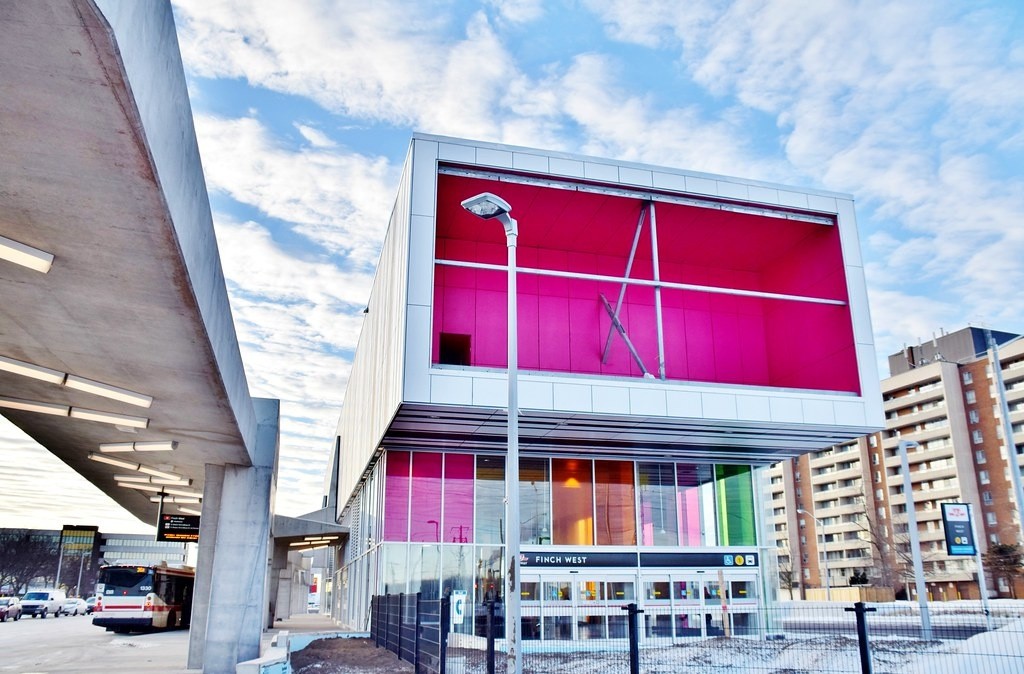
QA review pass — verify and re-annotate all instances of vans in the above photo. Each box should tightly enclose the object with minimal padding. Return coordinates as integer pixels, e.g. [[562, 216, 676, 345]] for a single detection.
[[19, 589, 67, 619]]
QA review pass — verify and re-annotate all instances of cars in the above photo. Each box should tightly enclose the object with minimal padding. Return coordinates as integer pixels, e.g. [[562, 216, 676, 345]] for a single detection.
[[60, 598, 88, 617], [86, 597, 96, 615], [0, 596, 22, 622]]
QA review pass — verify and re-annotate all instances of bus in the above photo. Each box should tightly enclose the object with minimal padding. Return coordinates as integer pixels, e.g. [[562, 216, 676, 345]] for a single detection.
[[91, 557, 195, 634]]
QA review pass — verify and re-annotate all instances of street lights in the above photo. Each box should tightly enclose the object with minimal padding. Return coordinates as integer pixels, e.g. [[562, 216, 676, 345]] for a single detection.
[[796, 509, 831, 602], [54, 541, 74, 589], [426, 520, 441, 553], [459, 191, 523, 674], [75, 552, 92, 597], [897, 440, 933, 640]]
